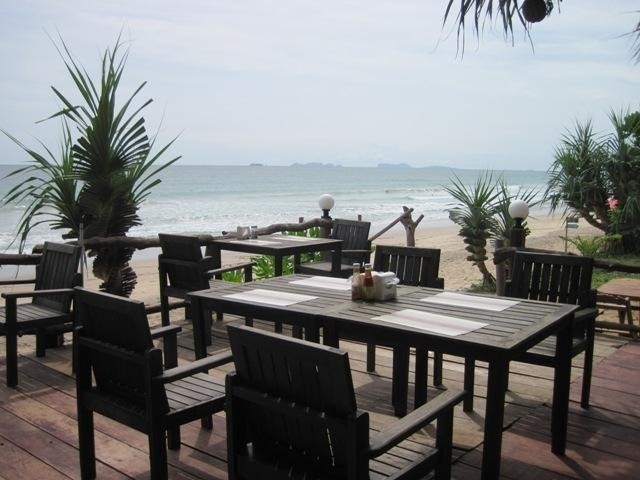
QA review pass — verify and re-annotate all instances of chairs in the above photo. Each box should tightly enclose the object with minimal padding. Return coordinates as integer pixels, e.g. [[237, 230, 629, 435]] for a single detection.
[[463, 245, 600, 414], [220, 320, 463, 478], [157, 218, 371, 346], [0, 240, 83, 388], [72, 284, 231, 478], [327, 245, 444, 419]]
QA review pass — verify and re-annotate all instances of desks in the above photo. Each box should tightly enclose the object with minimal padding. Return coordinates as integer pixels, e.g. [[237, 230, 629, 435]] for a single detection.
[[187, 270, 578, 476]]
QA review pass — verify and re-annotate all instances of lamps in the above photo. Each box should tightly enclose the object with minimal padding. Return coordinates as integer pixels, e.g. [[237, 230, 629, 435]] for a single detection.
[[507, 200, 530, 229], [316, 194, 335, 219]]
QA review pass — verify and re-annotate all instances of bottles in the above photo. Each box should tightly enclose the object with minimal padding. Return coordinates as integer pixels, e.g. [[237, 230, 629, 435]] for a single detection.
[[351, 263, 375, 302], [251, 226, 257, 239]]
